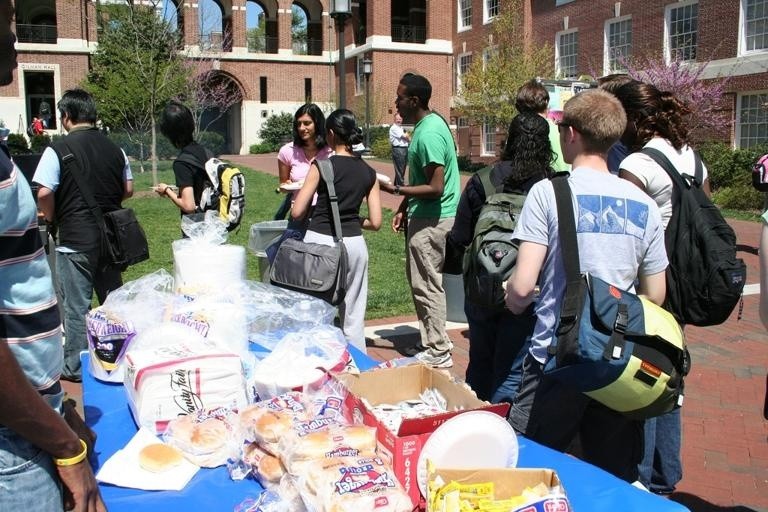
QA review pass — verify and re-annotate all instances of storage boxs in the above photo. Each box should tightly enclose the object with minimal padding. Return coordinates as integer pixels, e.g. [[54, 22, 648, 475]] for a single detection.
[[331, 364, 511, 511]]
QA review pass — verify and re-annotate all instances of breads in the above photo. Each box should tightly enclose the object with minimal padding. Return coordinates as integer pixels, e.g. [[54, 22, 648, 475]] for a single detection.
[[314, 459, 345, 481], [324, 491, 358, 512], [360, 452, 390, 474], [259, 454, 283, 477], [255, 411, 289, 439], [341, 425, 370, 451], [189, 418, 223, 456], [139, 444, 177, 470], [301, 431, 330, 457]]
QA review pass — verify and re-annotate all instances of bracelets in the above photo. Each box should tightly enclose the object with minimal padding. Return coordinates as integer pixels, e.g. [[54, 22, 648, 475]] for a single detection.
[[164, 185, 173, 193], [53, 438, 87, 466], [393, 184, 400, 196], [61, 390, 69, 405]]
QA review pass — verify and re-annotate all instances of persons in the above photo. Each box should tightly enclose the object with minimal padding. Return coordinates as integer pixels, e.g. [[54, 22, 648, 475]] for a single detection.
[[27, 113, 49, 140], [516, 72, 639, 179], [760, 199, 768, 423], [604, 82, 712, 497], [376, 72, 462, 368], [31, 88, 133, 381], [290, 108, 381, 355], [501, 87, 671, 486], [0, 0, 108, 512], [155, 103, 214, 239], [389, 113, 411, 186], [275, 103, 333, 242], [442, 114, 558, 399]]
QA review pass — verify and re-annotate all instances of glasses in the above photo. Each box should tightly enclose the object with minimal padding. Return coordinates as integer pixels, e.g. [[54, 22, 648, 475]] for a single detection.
[[295, 120, 313, 127], [555, 122, 569, 132]]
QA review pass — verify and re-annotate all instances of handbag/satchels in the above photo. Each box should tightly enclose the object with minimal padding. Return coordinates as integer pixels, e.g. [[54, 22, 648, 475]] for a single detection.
[[542, 276, 692, 419], [443, 234, 465, 275], [97, 207, 150, 271], [269, 238, 349, 306]]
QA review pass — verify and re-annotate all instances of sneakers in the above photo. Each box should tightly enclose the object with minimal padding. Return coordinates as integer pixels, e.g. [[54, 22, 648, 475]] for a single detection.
[[403, 346, 421, 356], [415, 351, 453, 368]]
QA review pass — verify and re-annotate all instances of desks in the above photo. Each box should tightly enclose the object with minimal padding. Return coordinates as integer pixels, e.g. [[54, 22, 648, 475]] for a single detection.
[[79, 325, 691, 512]]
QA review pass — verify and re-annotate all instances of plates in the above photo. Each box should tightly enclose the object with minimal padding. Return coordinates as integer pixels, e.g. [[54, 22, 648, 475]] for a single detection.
[[416, 410, 519, 501], [376, 171, 391, 184], [150, 184, 178, 192], [280, 181, 303, 191]]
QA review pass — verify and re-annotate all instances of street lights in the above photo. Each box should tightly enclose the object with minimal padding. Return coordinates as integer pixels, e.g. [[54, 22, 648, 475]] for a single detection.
[[361, 56, 374, 154], [329, 1, 357, 110]]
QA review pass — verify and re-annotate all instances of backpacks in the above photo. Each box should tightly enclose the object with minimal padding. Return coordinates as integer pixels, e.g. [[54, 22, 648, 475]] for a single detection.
[[752, 153, 768, 192], [638, 147, 759, 327], [177, 148, 246, 235], [462, 163, 539, 323]]
